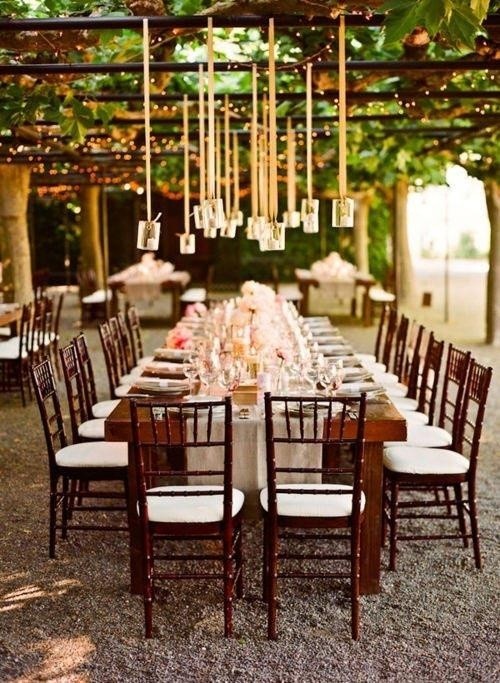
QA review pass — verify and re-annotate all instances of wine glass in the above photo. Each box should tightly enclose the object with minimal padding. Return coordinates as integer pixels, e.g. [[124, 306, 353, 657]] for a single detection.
[[185, 296, 344, 404]]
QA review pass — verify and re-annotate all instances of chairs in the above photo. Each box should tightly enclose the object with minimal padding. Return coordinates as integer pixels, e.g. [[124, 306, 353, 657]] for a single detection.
[[352, 302, 492, 571], [129, 396, 245, 640], [30, 306, 144, 560], [260, 391, 367, 639], [1, 293, 65, 409]]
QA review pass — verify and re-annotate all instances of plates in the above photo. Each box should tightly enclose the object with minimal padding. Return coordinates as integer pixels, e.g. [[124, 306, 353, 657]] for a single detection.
[[285, 390, 342, 418], [133, 344, 192, 396], [167, 401, 225, 418], [316, 335, 385, 401]]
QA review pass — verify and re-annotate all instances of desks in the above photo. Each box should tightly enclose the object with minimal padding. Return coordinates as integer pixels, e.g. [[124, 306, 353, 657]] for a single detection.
[[103, 313, 408, 595]]
